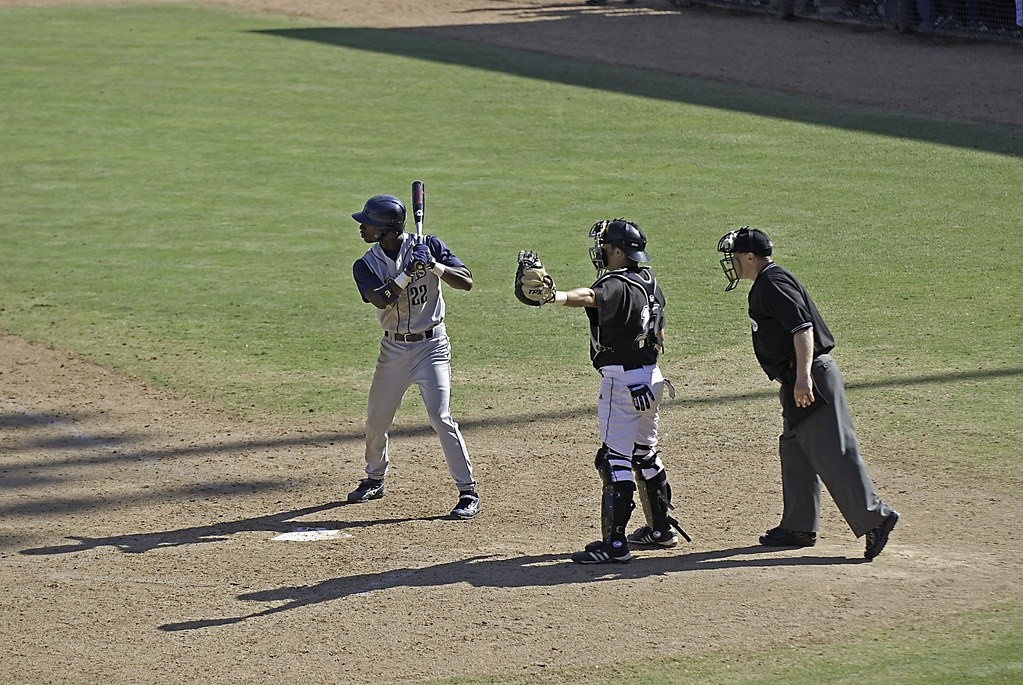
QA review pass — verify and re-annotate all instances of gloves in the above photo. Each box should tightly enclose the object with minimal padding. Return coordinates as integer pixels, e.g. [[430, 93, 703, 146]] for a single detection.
[[412, 245, 436, 270], [662, 378, 676, 400], [403, 250, 427, 277], [626, 384, 655, 411]]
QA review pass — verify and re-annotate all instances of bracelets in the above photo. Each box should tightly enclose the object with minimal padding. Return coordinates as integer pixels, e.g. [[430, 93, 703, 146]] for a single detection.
[[394, 271, 412, 290], [553, 291, 566, 305], [430, 263, 445, 278]]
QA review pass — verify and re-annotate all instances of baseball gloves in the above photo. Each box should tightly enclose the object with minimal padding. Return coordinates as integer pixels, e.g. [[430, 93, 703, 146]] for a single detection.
[[513, 248, 557, 311]]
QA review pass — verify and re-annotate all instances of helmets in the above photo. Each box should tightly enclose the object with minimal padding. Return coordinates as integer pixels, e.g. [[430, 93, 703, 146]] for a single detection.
[[350, 195, 407, 237], [717, 226, 773, 256], [588, 217, 652, 263]]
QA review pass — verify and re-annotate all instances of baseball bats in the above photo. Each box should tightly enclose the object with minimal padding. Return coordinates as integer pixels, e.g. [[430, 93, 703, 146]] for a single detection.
[[411, 179, 426, 271]]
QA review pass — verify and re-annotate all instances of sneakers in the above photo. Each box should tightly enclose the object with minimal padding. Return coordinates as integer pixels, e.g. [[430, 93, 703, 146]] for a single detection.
[[626, 526, 678, 548], [450, 492, 481, 519], [347, 478, 385, 503], [571, 542, 631, 565]]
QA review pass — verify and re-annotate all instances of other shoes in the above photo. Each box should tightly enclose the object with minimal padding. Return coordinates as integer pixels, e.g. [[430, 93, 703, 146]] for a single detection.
[[863, 509, 899, 560], [758, 527, 817, 549]]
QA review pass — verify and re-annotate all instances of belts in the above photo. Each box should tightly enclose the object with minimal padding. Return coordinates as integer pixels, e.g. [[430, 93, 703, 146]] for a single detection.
[[385, 329, 434, 344], [775, 377, 783, 384]]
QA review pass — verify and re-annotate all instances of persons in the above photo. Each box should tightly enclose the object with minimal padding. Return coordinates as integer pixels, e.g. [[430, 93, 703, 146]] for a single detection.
[[347, 194, 484, 520], [717, 226, 900, 557], [511, 218, 691, 564]]
